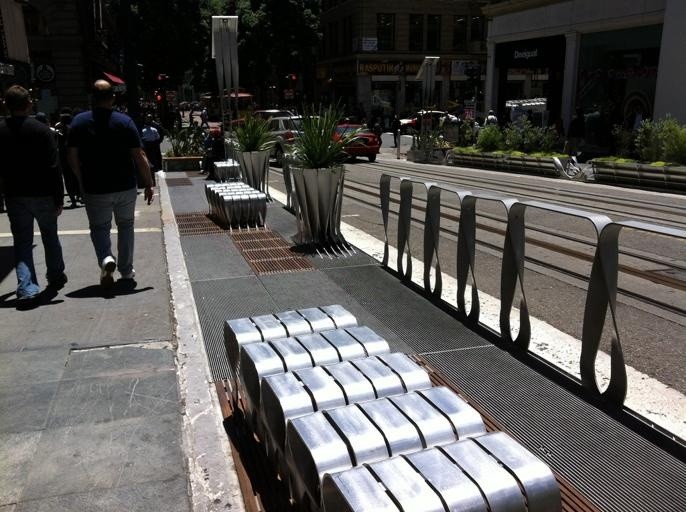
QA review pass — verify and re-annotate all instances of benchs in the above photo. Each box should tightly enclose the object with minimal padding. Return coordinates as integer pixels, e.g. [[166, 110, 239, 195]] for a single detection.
[[223, 303, 561, 512], [205, 181, 268, 233]]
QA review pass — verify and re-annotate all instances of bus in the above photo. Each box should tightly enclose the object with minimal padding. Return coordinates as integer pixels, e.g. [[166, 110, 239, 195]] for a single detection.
[[199, 91, 254, 122]]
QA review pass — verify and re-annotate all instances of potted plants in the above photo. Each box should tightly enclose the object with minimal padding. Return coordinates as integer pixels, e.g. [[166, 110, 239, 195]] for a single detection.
[[225, 99, 372, 250]]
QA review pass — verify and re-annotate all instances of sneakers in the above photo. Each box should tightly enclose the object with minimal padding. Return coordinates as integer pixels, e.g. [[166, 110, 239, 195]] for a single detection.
[[100, 256, 116, 285], [122, 268, 135, 279]]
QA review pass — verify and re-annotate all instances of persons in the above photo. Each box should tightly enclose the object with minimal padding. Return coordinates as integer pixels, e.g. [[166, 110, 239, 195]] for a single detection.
[[62, 78, 155, 291], [0, 84, 69, 310], [200, 106, 210, 128], [28, 102, 166, 209], [484, 109, 498, 126], [391, 113, 401, 148], [189, 109, 195, 127], [370, 116, 383, 154]]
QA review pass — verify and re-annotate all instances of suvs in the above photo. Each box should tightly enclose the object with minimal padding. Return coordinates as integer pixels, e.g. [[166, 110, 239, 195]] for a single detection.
[[399, 110, 461, 135]]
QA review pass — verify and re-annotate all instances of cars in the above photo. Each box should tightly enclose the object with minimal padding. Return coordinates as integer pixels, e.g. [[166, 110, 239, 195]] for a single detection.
[[251, 108, 381, 168]]
